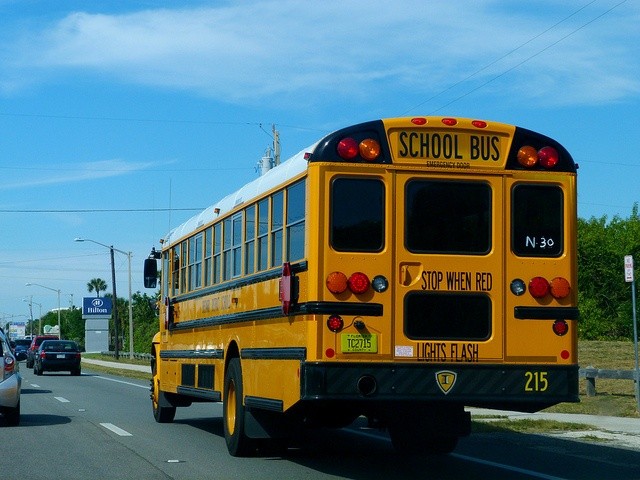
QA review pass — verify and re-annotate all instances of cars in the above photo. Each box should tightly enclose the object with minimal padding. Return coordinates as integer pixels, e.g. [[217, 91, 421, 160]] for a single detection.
[[15, 340, 32, 353], [0, 327, 27, 426], [33, 340, 81, 376]]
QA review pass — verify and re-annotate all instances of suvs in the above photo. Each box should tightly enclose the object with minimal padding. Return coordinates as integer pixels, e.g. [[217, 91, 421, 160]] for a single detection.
[[26, 336, 59, 368]]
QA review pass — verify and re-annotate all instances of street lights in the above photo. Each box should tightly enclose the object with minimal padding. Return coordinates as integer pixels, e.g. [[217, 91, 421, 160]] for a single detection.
[[24, 299, 41, 335], [75, 238, 134, 359], [26, 283, 60, 340]]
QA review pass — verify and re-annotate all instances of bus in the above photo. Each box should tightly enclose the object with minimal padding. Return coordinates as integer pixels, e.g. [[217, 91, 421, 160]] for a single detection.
[[144, 116, 579, 456]]
